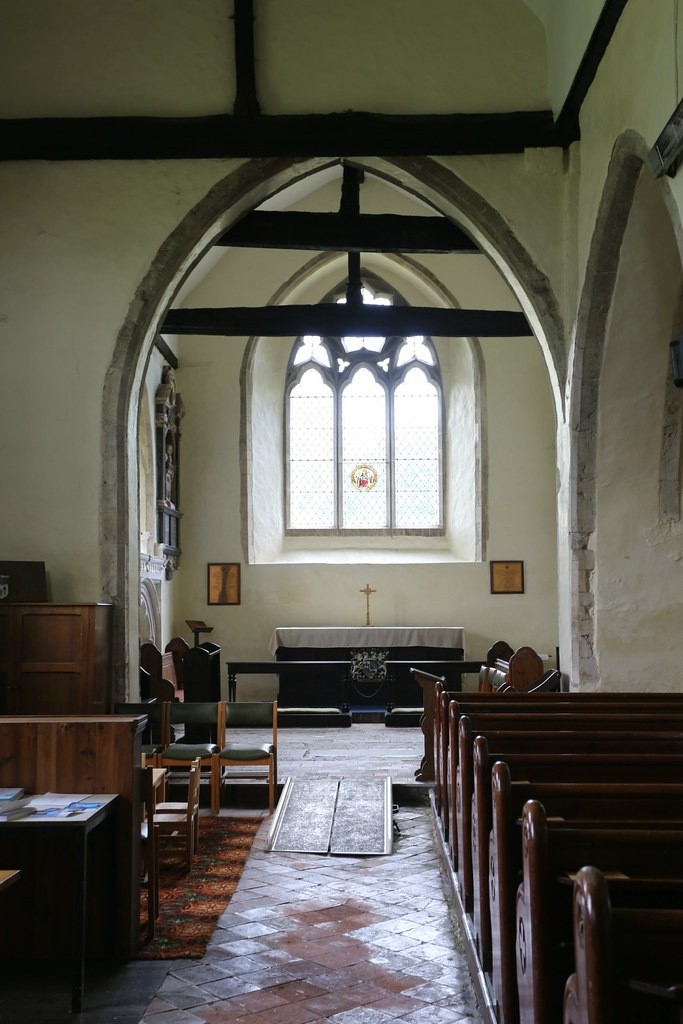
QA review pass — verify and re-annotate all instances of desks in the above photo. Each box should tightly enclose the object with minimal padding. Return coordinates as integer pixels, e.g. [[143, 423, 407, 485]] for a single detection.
[[0, 793, 119, 1013], [141, 767, 167, 873], [269, 626, 464, 708], [226, 659, 487, 704]]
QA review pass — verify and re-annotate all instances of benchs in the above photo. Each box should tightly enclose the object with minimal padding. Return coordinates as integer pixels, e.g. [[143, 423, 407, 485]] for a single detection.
[[429, 681, 683, 1023]]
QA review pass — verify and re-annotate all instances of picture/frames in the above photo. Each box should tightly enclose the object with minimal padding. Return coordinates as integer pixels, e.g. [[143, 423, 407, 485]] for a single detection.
[[207, 562, 241, 605], [490, 560, 524, 595]]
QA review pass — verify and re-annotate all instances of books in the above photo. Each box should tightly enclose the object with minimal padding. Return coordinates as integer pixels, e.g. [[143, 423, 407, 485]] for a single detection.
[[0, 787, 37, 823]]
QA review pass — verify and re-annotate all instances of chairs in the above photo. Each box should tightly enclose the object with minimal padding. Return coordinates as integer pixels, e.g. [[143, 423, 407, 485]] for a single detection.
[[478, 666, 507, 692], [111, 700, 277, 939]]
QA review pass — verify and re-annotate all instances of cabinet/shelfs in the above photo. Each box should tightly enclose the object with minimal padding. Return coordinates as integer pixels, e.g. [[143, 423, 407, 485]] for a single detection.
[[0, 600, 114, 718]]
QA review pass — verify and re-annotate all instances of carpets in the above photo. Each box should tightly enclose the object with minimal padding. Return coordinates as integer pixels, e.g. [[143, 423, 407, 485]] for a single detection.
[[137, 817, 265, 960]]
